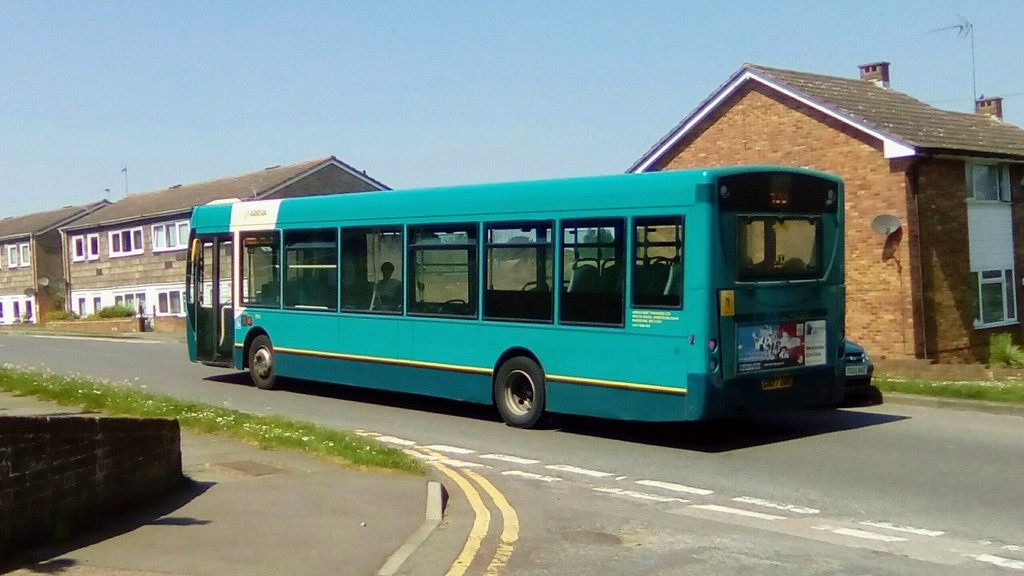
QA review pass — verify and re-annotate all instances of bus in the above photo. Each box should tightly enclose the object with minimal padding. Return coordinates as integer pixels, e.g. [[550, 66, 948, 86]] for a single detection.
[[184, 165, 845, 430]]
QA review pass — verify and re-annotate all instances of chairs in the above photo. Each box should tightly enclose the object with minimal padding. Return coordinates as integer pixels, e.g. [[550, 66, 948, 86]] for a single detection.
[[436, 299, 469, 317], [522, 255, 809, 292]]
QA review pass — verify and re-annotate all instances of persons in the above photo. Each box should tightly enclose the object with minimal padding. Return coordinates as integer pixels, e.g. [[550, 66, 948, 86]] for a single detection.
[[374, 262, 400, 309]]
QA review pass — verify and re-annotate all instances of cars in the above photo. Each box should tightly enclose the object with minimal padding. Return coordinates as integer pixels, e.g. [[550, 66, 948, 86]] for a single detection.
[[846, 339, 874, 394]]
